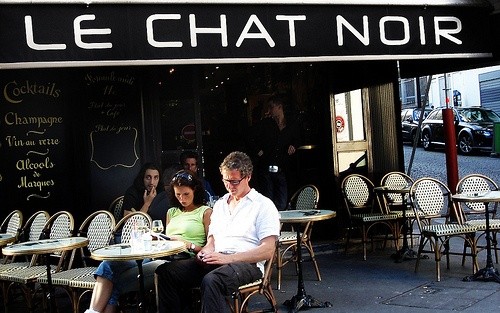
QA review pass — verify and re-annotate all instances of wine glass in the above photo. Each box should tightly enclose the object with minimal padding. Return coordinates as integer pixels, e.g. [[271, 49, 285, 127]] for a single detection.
[[152, 220, 164, 244]]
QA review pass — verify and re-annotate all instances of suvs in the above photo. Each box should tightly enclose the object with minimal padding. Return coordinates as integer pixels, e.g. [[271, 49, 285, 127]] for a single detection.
[[401, 106, 500, 156]]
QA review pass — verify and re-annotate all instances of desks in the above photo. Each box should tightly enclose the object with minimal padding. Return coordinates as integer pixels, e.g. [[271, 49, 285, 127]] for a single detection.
[[373, 185, 430, 264], [452, 191, 500, 283], [277, 208, 336, 313], [0, 234, 16, 247], [91, 240, 186, 313], [2, 237, 90, 313]]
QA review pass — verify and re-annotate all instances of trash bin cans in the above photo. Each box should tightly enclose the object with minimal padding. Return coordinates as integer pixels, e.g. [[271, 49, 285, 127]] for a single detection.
[[492, 121, 500, 154]]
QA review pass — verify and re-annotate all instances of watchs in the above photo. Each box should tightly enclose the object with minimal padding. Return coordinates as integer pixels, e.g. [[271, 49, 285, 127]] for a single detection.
[[188, 242, 195, 251]]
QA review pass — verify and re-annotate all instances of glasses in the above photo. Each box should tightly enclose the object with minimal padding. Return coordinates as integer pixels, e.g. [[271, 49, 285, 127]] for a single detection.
[[222, 176, 246, 185]]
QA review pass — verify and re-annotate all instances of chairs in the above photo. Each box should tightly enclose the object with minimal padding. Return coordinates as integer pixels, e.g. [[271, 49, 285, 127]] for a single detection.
[[0, 171, 500, 313]]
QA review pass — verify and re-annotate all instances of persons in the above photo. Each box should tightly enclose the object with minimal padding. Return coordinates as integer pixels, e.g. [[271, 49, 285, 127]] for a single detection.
[[252, 95, 301, 210], [154, 151, 281, 313], [179, 151, 216, 202], [114, 163, 170, 245], [82, 170, 213, 313]]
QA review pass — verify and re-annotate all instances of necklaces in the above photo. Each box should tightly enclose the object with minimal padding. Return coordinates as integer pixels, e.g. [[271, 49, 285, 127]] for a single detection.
[[234, 190, 250, 201]]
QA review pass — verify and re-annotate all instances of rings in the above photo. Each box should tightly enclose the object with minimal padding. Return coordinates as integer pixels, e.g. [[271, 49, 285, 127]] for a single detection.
[[153, 196, 155, 197]]
